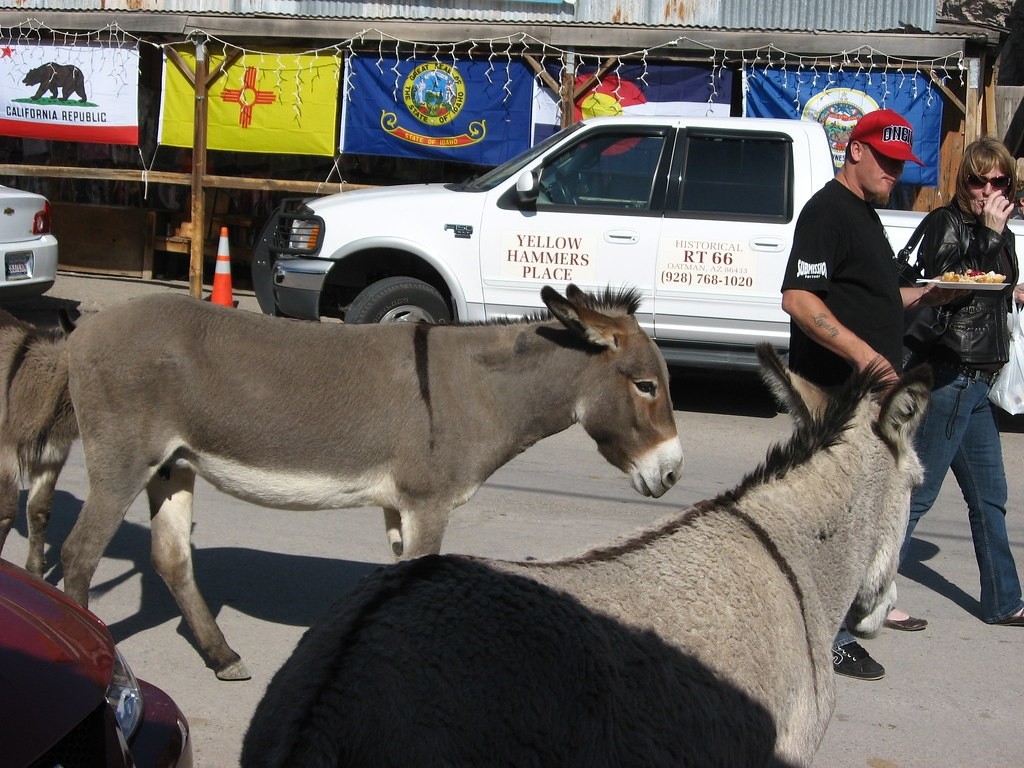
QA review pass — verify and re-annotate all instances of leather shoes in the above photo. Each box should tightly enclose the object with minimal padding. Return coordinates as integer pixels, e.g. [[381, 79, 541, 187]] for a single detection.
[[882, 614, 927, 631], [996, 606, 1024, 626]]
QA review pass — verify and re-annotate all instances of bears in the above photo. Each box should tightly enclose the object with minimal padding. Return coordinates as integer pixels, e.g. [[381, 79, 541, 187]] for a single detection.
[[22, 62, 87, 103]]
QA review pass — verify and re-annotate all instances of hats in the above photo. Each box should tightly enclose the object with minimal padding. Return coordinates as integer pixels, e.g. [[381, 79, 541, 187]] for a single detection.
[[849, 108, 925, 167]]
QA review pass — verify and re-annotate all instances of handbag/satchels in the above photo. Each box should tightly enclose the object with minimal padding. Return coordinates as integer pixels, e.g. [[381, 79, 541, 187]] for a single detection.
[[895, 252, 951, 355]]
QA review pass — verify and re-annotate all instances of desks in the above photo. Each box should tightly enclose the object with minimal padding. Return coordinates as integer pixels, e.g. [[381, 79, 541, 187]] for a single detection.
[[157, 236, 218, 255]]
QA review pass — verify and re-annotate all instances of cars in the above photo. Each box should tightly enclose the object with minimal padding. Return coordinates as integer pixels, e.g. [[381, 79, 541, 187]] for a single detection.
[[0, 185, 60, 304]]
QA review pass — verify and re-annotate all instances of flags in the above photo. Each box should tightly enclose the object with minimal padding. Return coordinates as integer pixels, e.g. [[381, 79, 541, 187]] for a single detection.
[[0, 45, 139, 145], [156, 48, 341, 157], [340, 51, 732, 166], [742, 69, 939, 189]]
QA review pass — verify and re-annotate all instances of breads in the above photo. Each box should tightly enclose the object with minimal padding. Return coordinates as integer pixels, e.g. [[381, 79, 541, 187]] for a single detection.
[[943, 269, 1007, 284]]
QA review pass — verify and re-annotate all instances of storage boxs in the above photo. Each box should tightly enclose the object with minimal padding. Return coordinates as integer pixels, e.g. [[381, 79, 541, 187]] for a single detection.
[[175, 192, 229, 240]]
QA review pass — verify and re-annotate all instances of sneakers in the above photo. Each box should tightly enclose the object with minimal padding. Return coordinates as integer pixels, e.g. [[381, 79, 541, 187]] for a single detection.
[[831, 642, 884, 681]]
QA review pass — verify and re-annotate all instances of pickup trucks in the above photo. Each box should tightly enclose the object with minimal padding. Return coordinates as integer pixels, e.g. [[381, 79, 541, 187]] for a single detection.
[[253, 117, 1024, 413]]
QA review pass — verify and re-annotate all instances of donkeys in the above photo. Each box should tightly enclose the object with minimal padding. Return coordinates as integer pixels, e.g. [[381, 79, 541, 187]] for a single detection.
[[240, 340, 927, 768], [18, 283, 683, 683], [0, 302, 79, 577]]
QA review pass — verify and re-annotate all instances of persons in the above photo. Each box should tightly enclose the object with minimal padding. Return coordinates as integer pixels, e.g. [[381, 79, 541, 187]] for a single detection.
[[884, 138, 1024, 632], [780, 109, 953, 681]]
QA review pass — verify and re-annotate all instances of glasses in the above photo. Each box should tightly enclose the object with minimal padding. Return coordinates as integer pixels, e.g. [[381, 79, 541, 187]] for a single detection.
[[966, 174, 1010, 190]]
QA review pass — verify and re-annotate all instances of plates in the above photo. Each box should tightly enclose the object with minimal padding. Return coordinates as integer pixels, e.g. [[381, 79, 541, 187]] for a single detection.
[[928, 281, 1011, 290]]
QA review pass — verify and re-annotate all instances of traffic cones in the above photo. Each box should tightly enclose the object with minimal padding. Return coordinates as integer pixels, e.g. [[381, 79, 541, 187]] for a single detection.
[[209, 223, 240, 309]]
